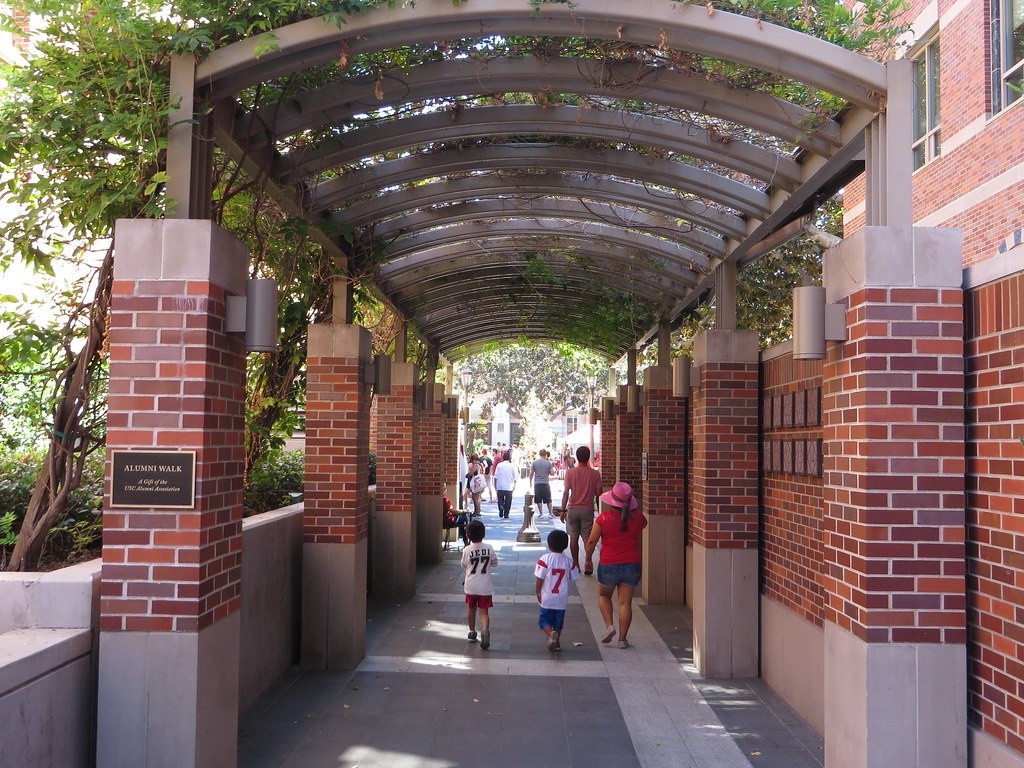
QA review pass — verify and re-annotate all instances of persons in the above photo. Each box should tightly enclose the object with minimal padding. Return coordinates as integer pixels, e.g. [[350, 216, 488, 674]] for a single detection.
[[585, 482, 648, 649], [534, 530, 580, 651], [460, 521, 498, 650], [444, 484, 470, 546], [460, 441, 601, 520], [560, 446, 602, 574]]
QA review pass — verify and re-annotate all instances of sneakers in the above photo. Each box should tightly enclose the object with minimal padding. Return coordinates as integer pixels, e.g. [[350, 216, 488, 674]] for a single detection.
[[538, 514, 544, 518], [480, 629, 490, 649], [601, 626, 616, 644], [467, 631, 477, 642], [548, 631, 561, 651], [548, 514, 556, 518], [618, 640, 630, 649]]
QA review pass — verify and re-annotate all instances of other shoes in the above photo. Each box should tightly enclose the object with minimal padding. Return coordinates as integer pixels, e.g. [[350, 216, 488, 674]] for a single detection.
[[471, 512, 482, 519], [499, 515, 509, 518]]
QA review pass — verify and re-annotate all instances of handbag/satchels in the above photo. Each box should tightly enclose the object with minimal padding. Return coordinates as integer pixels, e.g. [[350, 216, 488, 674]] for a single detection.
[[470, 475, 488, 493]]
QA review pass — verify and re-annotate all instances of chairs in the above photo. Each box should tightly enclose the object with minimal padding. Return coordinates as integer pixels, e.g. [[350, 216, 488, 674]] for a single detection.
[[442, 498, 472, 551]]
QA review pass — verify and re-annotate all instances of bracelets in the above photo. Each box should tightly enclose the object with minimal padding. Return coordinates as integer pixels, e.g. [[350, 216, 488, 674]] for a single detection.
[[561, 509, 567, 512]]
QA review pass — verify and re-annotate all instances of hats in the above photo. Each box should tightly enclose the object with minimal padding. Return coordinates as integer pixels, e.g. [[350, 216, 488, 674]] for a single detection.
[[599, 482, 638, 510], [481, 449, 487, 455]]
